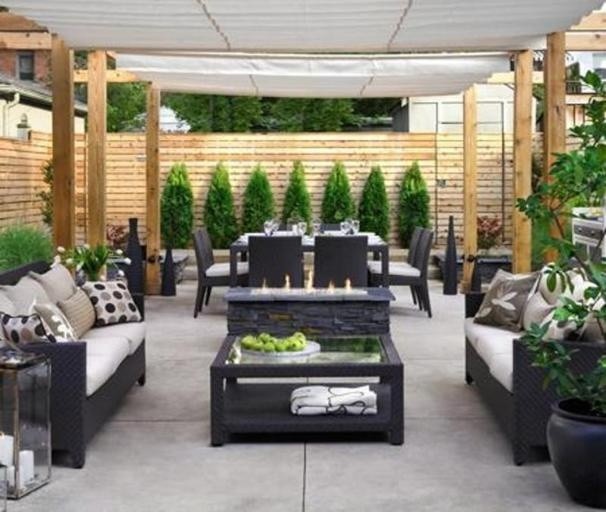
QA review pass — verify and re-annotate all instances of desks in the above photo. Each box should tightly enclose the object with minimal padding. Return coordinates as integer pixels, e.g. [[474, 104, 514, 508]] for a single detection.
[[0, 349, 55, 502]]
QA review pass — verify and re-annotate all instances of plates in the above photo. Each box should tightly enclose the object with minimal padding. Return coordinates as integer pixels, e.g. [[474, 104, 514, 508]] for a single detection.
[[242, 340, 321, 358]]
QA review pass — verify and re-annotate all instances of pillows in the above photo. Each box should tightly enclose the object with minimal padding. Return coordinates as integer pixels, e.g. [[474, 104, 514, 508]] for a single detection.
[[0, 254, 143, 347], [474, 257, 606, 342]]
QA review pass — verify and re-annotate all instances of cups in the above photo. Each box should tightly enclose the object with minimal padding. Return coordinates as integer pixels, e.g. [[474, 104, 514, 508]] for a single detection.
[[313, 223, 321, 231], [292, 222, 307, 236], [340, 218, 360, 236]]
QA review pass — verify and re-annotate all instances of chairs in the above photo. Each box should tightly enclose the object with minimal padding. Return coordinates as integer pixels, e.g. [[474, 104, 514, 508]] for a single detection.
[[190, 225, 436, 319]]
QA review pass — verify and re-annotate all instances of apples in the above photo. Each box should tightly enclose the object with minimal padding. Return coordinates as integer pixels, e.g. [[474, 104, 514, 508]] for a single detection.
[[242, 332, 306, 352]]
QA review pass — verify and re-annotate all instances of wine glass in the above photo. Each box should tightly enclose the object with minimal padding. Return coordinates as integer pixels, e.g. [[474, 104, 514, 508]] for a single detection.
[[264, 218, 279, 236]]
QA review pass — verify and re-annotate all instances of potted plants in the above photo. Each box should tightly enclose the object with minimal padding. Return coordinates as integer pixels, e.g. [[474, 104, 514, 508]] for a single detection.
[[516, 177, 606, 510]]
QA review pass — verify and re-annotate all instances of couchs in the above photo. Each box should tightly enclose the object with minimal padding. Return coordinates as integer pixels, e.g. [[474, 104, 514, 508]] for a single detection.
[[458, 256, 606, 511], [0, 260, 145, 471]]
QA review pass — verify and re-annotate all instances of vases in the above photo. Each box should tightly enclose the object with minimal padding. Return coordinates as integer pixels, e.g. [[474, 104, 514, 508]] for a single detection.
[[160, 244, 176, 296], [123, 218, 143, 292]]
